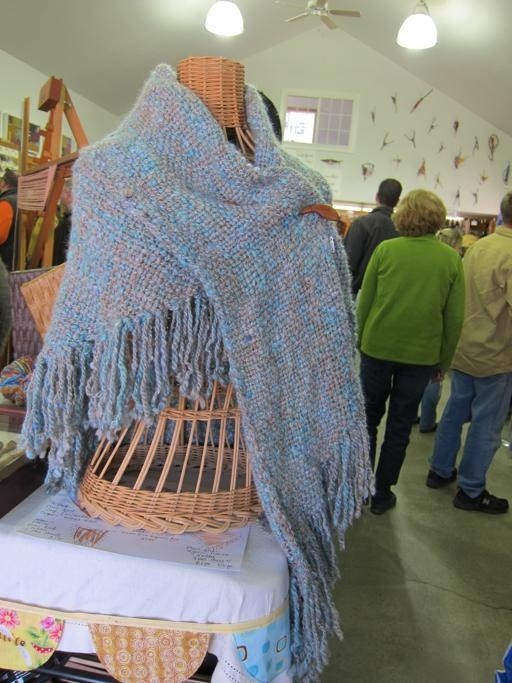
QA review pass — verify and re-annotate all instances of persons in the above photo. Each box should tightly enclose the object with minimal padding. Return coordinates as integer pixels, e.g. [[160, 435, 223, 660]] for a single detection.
[[424, 190, 511, 514], [410, 228, 465, 433], [352, 186, 467, 515], [52, 211, 74, 263], [1, 168, 20, 271], [27, 202, 63, 270], [341, 177, 404, 304]]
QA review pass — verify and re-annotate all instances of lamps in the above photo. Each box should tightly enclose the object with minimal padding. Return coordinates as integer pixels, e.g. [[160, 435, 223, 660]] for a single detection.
[[396, 0, 438, 50], [204, 0, 244, 37]]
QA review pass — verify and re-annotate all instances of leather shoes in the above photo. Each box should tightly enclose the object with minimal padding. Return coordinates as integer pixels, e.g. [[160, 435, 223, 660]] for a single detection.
[[453, 490, 509, 514], [426, 468, 457, 488], [371, 491, 396, 514], [413, 416, 437, 432]]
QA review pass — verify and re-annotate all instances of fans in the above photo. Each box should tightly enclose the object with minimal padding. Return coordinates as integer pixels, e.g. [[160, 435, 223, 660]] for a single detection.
[[273, 0, 360, 30]]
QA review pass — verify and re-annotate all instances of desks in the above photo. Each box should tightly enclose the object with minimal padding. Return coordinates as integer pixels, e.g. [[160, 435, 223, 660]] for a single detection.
[[0, 466, 328, 683]]
